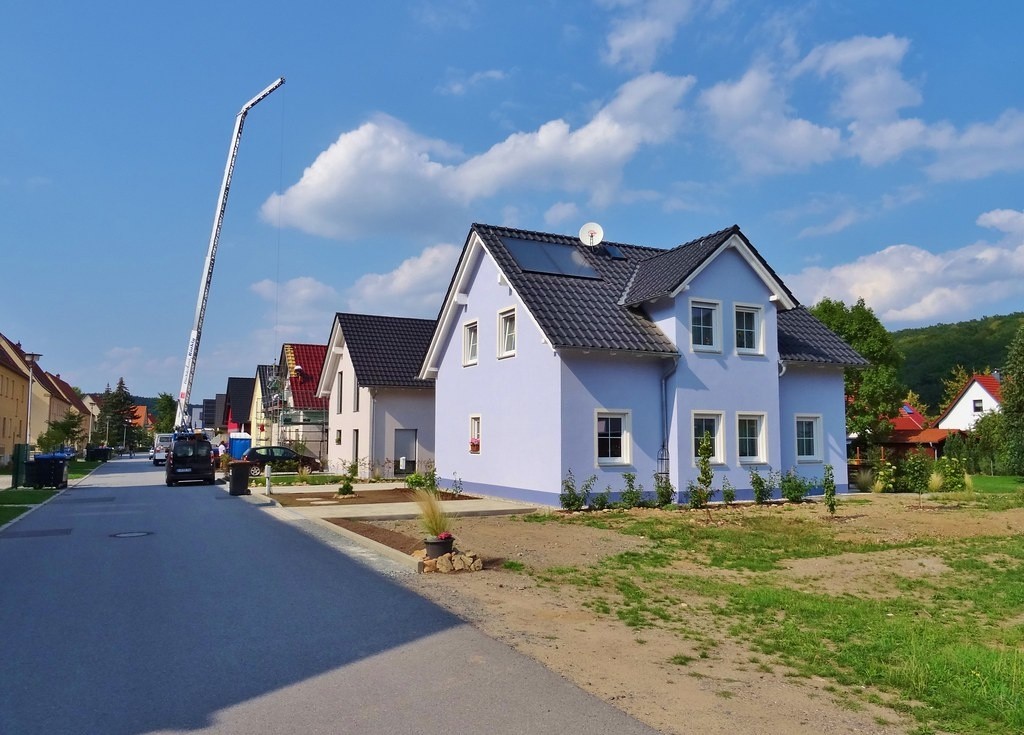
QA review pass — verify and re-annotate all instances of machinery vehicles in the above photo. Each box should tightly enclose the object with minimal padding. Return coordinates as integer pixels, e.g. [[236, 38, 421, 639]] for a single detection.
[[173, 76, 286, 451]]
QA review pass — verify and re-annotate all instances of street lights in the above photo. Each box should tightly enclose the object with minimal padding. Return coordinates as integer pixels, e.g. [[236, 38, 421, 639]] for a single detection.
[[25, 353, 42, 444], [106, 416, 112, 447], [89, 402, 95, 443]]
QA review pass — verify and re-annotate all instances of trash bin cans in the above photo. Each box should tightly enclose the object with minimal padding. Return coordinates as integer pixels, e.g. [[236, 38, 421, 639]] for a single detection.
[[36, 450, 71, 488], [225, 460, 252, 496]]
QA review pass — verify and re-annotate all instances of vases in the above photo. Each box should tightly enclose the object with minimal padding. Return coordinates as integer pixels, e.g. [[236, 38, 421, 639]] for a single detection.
[[471, 438, 480, 444], [471, 445, 479, 451]]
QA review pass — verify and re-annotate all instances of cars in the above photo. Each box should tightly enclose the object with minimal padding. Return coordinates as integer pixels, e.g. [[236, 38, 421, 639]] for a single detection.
[[241, 446, 323, 477]]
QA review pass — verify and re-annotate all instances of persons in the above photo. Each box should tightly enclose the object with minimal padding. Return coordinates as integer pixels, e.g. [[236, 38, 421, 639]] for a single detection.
[[218, 441, 226, 469], [129, 443, 133, 458]]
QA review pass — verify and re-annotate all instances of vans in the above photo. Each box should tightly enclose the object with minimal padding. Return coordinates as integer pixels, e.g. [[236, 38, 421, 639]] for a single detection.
[[164, 438, 217, 487], [151, 433, 175, 467]]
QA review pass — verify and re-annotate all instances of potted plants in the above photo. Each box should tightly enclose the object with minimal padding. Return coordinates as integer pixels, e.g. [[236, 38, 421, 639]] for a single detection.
[[403, 486, 455, 560]]
